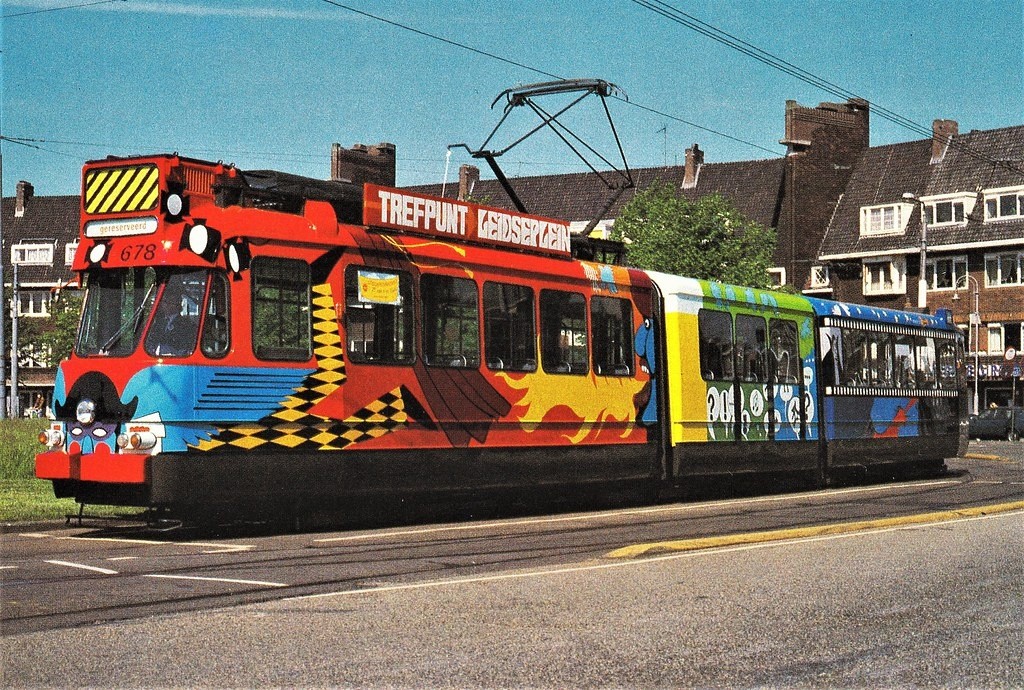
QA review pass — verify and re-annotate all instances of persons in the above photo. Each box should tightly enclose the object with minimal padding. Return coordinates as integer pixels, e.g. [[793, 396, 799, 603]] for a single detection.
[[147, 278, 203, 352]]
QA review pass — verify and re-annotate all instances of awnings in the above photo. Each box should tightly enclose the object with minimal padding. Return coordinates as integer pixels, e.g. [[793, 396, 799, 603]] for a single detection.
[[936, 355, 1024, 381]]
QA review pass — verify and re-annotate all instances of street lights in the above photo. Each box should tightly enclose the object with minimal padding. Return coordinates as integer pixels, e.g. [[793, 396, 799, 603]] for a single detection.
[[953, 275, 985, 416], [900, 192, 928, 308]]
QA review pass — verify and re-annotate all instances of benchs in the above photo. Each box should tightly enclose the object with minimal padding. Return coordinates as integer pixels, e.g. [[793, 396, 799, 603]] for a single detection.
[[257, 345, 368, 362]]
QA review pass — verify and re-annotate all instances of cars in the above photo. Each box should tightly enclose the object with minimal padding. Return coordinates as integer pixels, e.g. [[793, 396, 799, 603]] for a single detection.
[[969, 406, 1024, 441]]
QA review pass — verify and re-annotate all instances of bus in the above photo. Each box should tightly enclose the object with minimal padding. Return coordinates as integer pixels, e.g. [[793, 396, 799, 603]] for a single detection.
[[33, 77, 971, 535]]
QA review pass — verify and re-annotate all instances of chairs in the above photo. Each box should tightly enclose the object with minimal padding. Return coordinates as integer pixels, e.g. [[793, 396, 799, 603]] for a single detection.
[[466, 356, 504, 369], [701, 369, 715, 380], [600, 363, 629, 375], [756, 374, 779, 383], [869, 378, 886, 388], [502, 358, 538, 371], [739, 372, 758, 383], [429, 354, 467, 367], [568, 362, 602, 374], [543, 361, 571, 373], [898, 379, 916, 389], [922, 380, 942, 389], [776, 375, 798, 384], [713, 370, 735, 380], [883, 379, 901, 388], [838, 377, 857, 387]]
